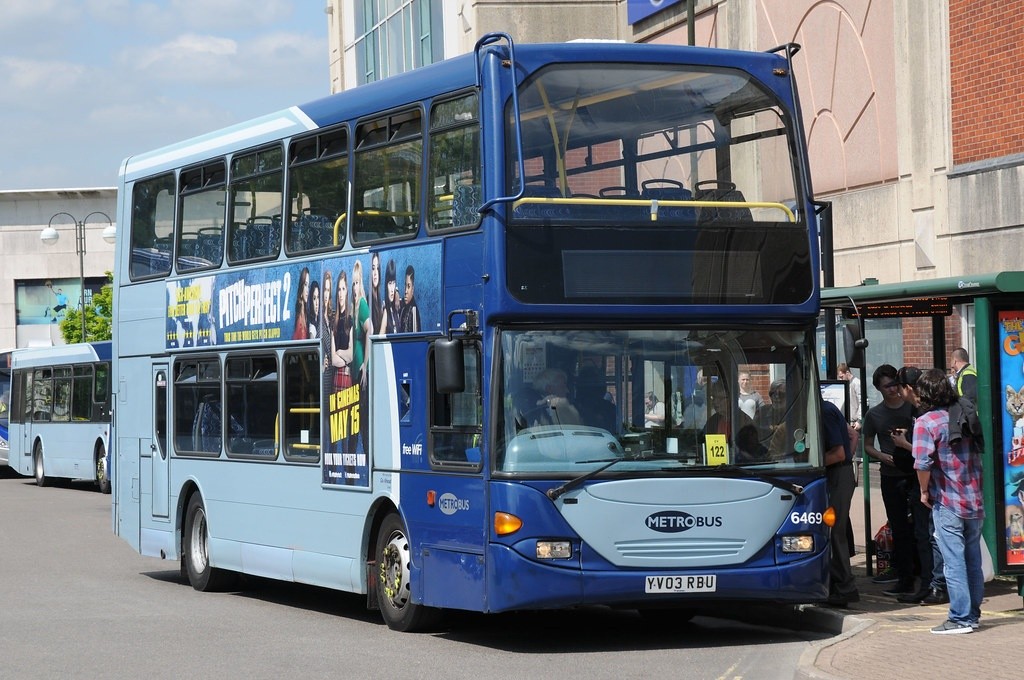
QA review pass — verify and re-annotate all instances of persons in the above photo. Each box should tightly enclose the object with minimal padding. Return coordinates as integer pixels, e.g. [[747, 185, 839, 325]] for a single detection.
[[886, 366, 987, 635], [948, 348, 977, 407], [820, 400, 868, 603], [836, 363, 861, 486], [752, 378, 786, 449], [768, 421, 787, 462], [643, 391, 664, 428], [702, 380, 756, 465], [524, 367, 580, 425], [291, 253, 421, 485], [694, 368, 708, 402], [864, 364, 916, 595], [738, 370, 765, 418]]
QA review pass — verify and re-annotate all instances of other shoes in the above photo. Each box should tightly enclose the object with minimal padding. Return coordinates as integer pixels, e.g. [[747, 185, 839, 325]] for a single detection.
[[882, 585, 915, 595], [921, 588, 950, 606], [897, 592, 928, 604], [846, 589, 860, 601], [819, 598, 848, 609]]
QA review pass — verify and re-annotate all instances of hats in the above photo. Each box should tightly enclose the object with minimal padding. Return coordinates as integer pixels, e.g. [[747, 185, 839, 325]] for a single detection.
[[888, 366, 923, 387]]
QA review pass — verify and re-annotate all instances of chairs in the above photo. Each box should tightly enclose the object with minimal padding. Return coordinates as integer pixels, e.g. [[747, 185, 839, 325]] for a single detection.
[[149, 175, 753, 278], [502, 356, 618, 435], [190, 390, 317, 456]]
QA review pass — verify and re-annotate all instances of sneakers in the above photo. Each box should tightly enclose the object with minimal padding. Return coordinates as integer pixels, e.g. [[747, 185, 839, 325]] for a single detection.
[[870, 567, 900, 583], [970, 618, 978, 629], [930, 619, 973, 634]]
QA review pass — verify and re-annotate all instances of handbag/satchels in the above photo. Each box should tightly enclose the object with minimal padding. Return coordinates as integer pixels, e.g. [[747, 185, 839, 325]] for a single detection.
[[874, 522, 894, 572], [979, 534, 995, 582]]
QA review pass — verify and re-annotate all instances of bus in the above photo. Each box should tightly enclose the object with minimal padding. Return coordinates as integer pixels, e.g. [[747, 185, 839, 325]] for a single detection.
[[110, 32, 870, 632], [0, 347, 34, 467], [7, 338, 112, 495]]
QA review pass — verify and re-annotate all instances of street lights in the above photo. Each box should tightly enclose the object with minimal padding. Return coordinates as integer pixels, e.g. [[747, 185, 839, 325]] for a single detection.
[[41, 210, 116, 344]]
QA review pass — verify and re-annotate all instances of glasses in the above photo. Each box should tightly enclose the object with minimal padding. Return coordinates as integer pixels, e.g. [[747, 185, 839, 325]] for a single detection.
[[769, 391, 787, 399], [710, 395, 729, 401]]
